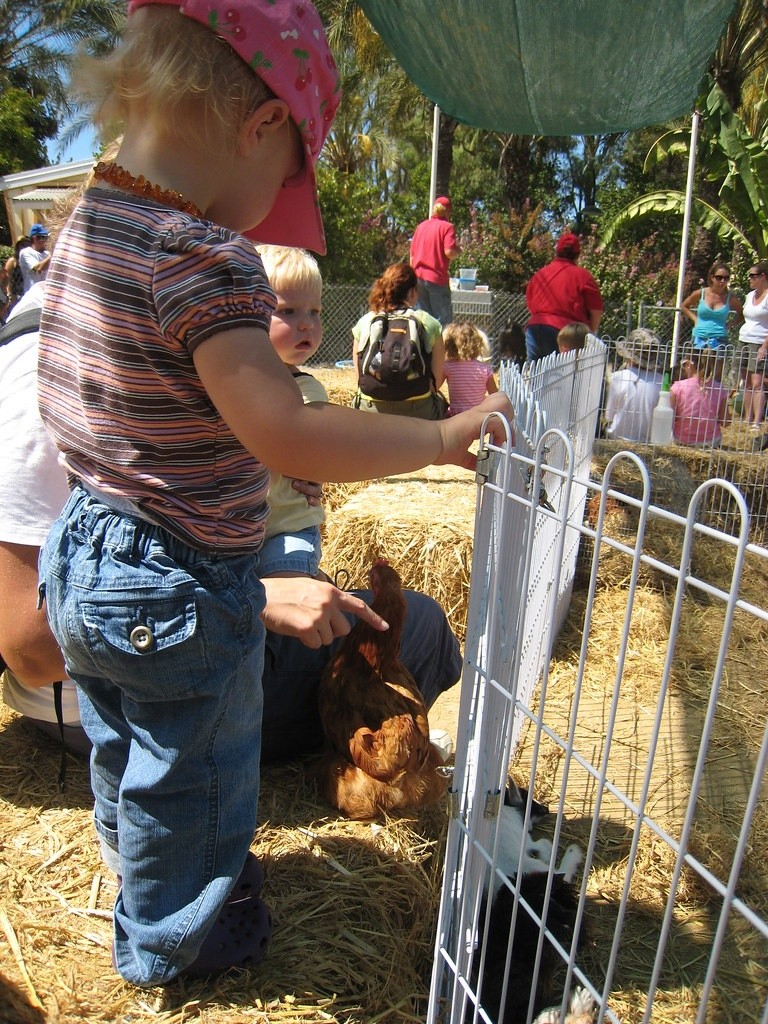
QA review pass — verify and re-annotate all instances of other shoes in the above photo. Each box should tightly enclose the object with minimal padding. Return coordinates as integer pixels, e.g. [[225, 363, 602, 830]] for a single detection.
[[427, 727, 453, 762]]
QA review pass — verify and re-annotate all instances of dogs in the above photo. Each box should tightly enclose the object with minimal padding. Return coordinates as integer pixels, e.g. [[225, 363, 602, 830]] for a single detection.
[[459, 773, 599, 1024]]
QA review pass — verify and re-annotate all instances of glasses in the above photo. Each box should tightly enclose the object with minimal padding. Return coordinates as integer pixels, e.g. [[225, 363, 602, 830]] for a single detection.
[[714, 274, 730, 283], [680, 359, 694, 369], [749, 273, 759, 279]]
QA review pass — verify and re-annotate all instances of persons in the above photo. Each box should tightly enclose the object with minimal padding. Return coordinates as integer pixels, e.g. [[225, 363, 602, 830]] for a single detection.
[[681, 264, 744, 383], [353, 264, 449, 418], [253, 242, 330, 684], [738, 262, 768, 432], [525, 235, 603, 370], [0, 139, 463, 778], [443, 323, 499, 418], [0, 225, 52, 325], [558, 323, 607, 438], [38, 0, 515, 988], [671, 350, 732, 448], [410, 197, 461, 330], [605, 328, 662, 444]]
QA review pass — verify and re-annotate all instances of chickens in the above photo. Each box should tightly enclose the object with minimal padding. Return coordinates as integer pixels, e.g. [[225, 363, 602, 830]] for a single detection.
[[316, 559, 448, 821]]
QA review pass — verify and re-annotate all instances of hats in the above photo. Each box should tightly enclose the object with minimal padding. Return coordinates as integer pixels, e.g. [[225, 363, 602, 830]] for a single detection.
[[434, 197, 454, 209], [129, 0, 343, 257], [617, 328, 660, 368], [30, 225, 49, 237], [557, 235, 580, 254]]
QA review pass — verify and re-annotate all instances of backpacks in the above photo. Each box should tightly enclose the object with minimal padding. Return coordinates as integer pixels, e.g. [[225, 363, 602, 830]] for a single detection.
[[357, 313, 432, 401]]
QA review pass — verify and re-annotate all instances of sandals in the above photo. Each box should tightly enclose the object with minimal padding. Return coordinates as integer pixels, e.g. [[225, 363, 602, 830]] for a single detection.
[[166, 898, 270, 981], [117, 851, 263, 903]]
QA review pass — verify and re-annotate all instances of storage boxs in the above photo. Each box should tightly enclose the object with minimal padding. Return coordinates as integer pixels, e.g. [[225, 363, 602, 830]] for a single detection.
[[459, 268, 477, 279], [475, 284, 490, 291], [459, 278, 477, 290]]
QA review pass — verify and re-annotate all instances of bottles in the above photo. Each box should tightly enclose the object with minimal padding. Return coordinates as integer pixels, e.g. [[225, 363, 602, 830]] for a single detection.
[[650, 374, 674, 446]]
